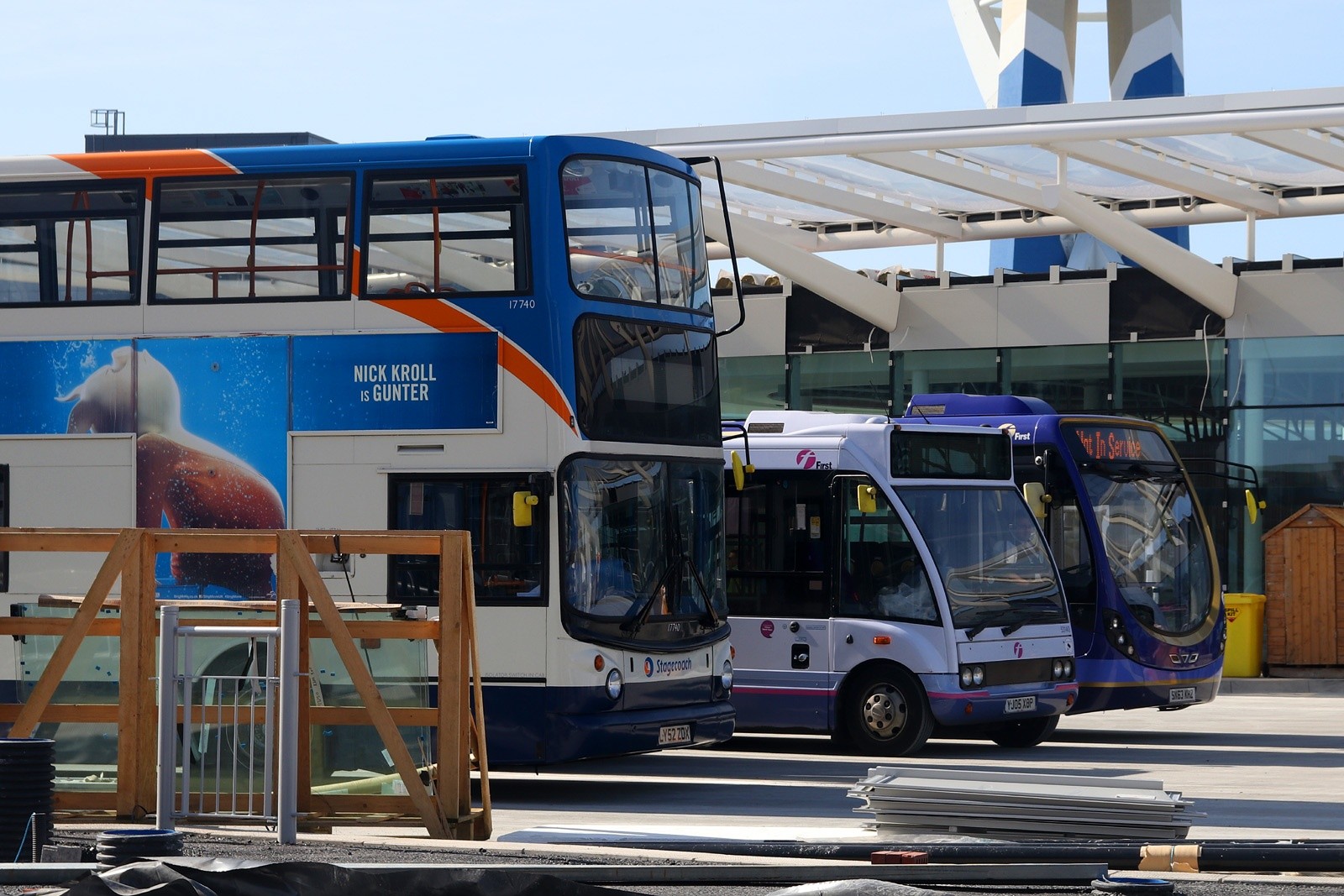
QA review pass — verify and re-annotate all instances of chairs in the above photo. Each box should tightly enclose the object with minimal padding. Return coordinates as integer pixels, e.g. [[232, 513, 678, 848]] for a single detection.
[[389, 282, 455, 293], [851, 540, 910, 589]]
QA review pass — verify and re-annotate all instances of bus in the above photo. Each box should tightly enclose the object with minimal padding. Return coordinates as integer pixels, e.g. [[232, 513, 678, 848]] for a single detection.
[[0, 136, 754, 774], [716, 423, 1080, 759], [722, 393, 1266, 749]]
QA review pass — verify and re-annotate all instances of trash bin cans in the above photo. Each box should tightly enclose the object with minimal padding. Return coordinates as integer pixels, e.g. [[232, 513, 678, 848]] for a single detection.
[[1222, 594, 1267, 677]]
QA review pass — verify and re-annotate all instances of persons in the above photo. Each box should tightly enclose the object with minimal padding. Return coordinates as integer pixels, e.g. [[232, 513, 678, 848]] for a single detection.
[[991, 512, 1044, 566]]
